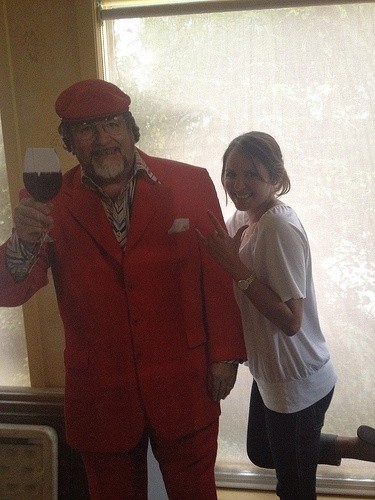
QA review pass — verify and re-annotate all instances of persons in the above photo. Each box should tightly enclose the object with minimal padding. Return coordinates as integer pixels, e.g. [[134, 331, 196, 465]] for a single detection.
[[193, 131, 375, 500], [0, 79, 248, 500]]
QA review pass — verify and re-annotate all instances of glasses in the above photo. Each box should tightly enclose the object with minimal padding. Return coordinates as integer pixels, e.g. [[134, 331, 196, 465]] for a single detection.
[[68, 115, 128, 141]]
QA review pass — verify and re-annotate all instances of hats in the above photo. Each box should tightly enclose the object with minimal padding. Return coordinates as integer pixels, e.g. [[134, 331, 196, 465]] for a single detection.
[[54, 79, 131, 120]]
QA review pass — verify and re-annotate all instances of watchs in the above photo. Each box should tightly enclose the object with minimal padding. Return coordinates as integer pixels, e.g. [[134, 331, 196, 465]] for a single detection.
[[237, 272, 257, 291]]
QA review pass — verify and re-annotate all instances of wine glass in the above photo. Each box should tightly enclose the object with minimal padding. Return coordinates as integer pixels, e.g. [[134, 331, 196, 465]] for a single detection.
[[22, 146, 62, 242]]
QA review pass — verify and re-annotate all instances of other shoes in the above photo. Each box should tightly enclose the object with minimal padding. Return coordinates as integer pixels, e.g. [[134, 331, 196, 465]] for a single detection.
[[356, 426, 375, 445]]
